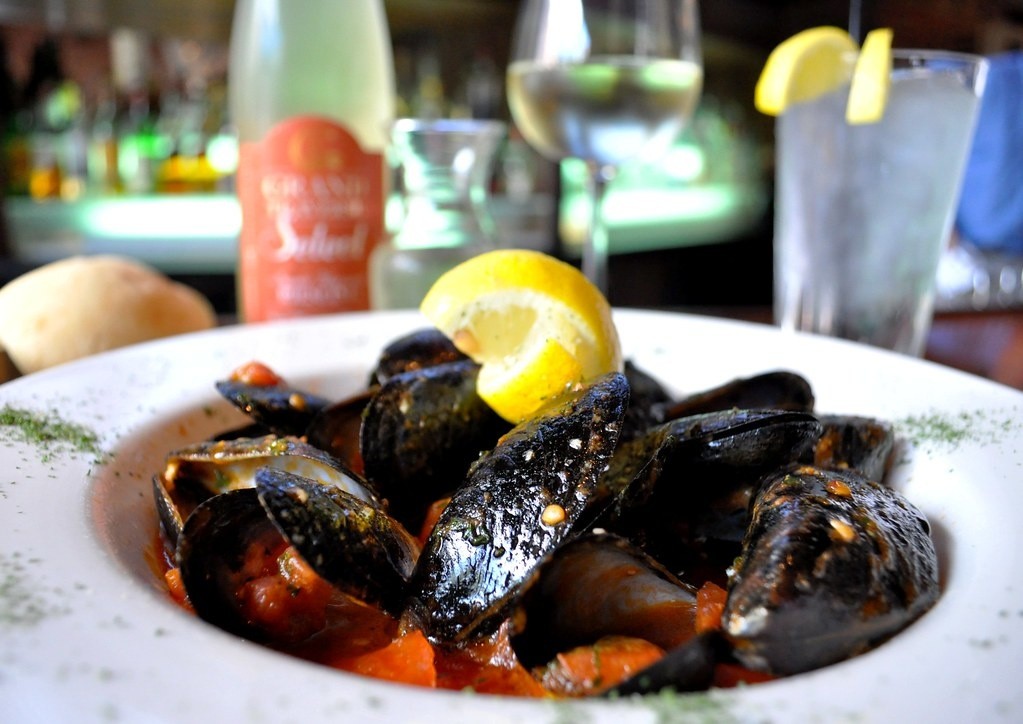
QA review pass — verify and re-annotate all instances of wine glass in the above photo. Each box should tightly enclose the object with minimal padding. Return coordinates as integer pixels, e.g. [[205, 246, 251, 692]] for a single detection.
[[508, 0, 704, 293], [920, 47, 1022, 374]]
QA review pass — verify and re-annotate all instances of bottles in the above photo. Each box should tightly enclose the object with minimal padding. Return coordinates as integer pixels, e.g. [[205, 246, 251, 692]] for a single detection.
[[226, 0, 395, 325], [102, 26, 162, 132], [388, 48, 509, 120], [368, 115, 509, 308]]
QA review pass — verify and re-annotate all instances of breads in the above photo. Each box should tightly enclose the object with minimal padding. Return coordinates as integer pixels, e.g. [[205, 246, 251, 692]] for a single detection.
[[0, 254, 217, 375]]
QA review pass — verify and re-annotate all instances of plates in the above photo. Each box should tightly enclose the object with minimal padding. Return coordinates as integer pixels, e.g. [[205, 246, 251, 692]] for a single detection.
[[0, 303, 1023, 724]]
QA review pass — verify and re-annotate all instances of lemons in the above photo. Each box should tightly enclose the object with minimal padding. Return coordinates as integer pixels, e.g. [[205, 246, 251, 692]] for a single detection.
[[473, 339, 586, 423], [753, 26, 860, 117], [845, 28, 894, 125], [420, 249, 622, 377]]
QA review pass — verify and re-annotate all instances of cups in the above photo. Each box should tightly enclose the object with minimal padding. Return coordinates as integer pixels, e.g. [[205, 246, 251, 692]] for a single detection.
[[773, 48, 988, 359]]
